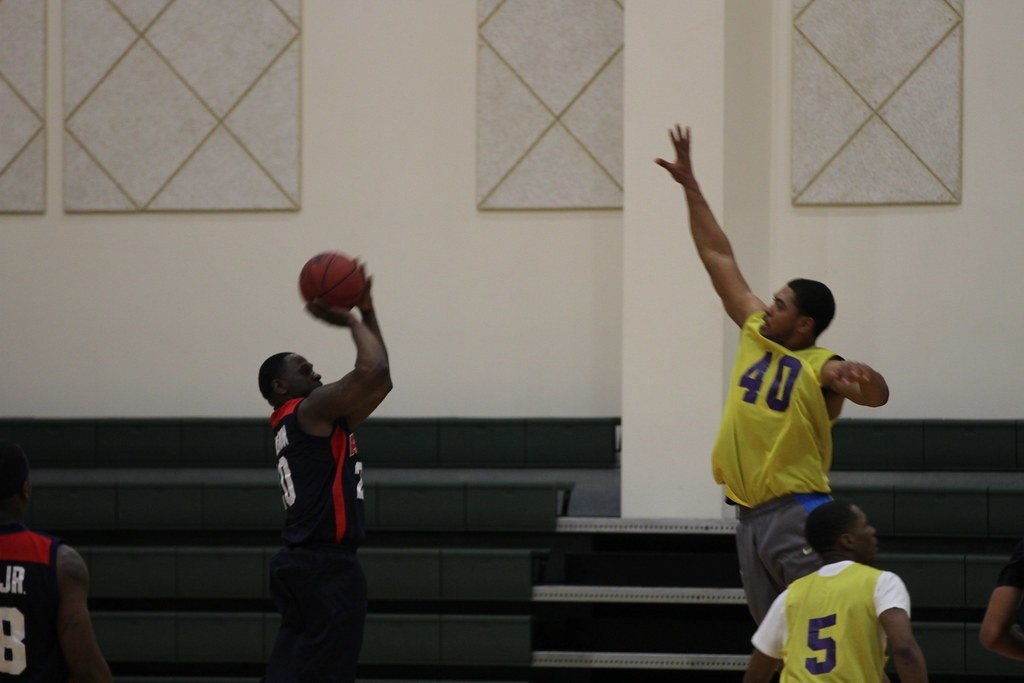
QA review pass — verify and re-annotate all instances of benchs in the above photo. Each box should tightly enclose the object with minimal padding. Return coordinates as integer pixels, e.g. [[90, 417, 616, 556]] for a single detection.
[[0, 415, 1024, 683]]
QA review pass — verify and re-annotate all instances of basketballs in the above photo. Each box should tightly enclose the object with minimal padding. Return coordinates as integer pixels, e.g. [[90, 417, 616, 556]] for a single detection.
[[297, 251, 364, 312]]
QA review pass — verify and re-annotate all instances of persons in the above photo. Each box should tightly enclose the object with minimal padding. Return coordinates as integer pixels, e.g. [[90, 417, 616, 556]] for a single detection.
[[743, 499, 928, 683], [978, 538, 1024, 661], [0, 439, 112, 683], [655, 123, 888, 628], [258, 261, 394, 683]]
